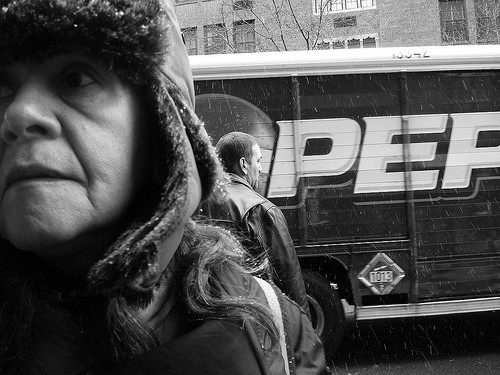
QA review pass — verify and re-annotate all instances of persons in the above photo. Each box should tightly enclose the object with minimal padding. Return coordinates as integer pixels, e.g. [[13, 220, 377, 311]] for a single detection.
[[210, 131, 312, 330], [0, 1, 331, 375]]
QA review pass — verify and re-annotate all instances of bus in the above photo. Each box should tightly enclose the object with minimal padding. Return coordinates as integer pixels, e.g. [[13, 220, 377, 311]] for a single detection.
[[188, 46, 500, 344]]
